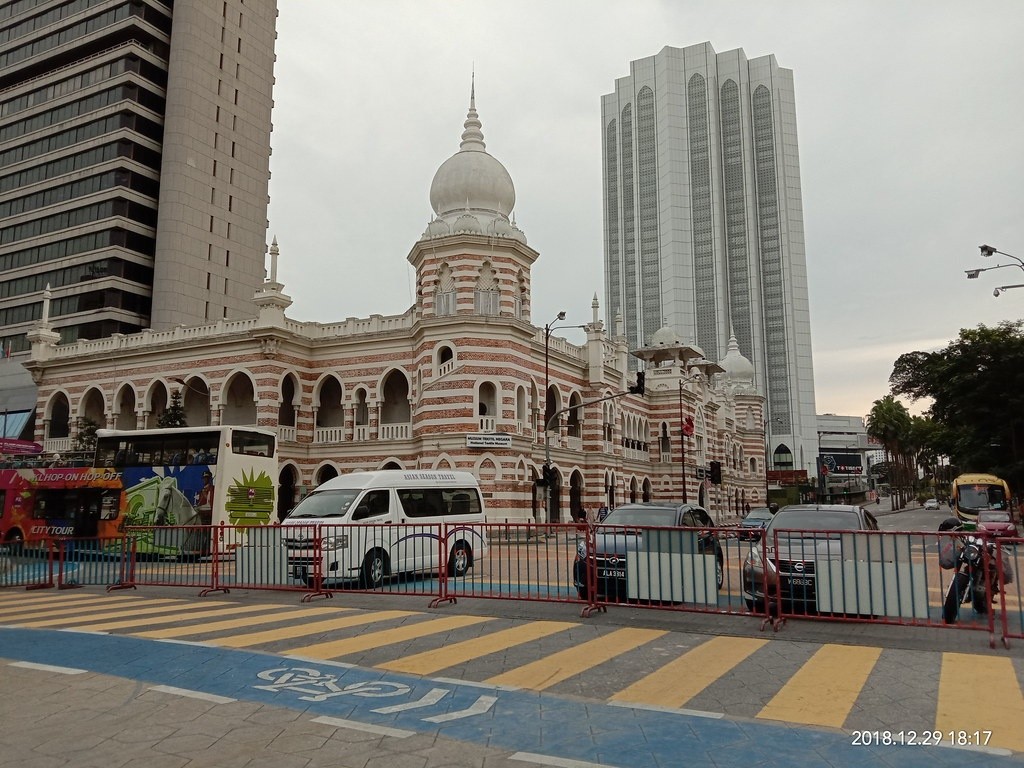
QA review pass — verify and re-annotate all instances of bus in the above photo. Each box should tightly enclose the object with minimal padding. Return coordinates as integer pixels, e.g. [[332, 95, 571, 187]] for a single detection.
[[0, 425, 278, 563], [950, 473, 1011, 524]]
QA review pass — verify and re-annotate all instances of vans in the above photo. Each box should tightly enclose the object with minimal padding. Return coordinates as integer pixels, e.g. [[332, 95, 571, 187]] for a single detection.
[[281, 469, 488, 588]]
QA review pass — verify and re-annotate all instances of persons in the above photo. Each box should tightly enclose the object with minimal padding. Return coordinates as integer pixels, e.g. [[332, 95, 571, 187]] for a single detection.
[[578, 505, 586, 520], [595, 502, 608, 522], [0, 444, 218, 469], [193, 470, 215, 556], [586, 505, 595, 525]]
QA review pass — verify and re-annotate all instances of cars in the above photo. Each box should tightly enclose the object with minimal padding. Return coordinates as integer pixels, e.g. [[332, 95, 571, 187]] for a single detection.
[[737, 509, 773, 541], [573, 502, 724, 606], [973, 511, 1018, 545], [924, 499, 940, 510], [743, 504, 880, 619]]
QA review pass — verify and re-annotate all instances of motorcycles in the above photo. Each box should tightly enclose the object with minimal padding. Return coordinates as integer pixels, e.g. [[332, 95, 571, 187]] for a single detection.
[[938, 518, 1013, 624]]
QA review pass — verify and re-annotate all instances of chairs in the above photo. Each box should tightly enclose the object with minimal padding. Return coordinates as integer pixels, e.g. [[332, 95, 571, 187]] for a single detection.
[[115, 450, 217, 464], [0, 457, 94, 467]]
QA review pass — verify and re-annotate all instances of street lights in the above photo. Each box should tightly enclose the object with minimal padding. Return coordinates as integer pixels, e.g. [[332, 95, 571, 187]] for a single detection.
[[545, 311, 592, 538], [764, 418, 783, 508], [679, 373, 713, 504], [846, 444, 855, 492]]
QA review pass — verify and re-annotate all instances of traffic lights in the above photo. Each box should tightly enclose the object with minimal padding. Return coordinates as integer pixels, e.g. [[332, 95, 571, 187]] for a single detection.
[[844, 487, 847, 494], [542, 465, 551, 487], [629, 370, 645, 397]]
